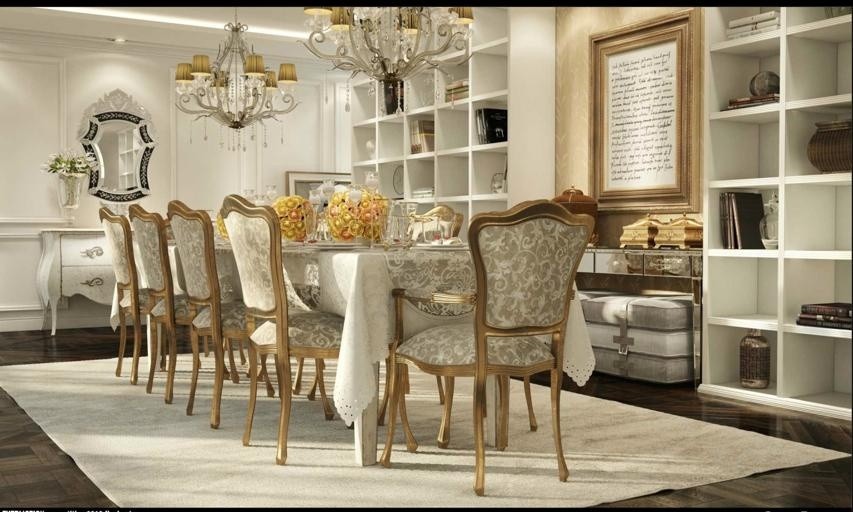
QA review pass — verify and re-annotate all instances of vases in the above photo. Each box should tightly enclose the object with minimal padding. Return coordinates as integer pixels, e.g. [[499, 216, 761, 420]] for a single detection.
[[55, 171, 83, 210]]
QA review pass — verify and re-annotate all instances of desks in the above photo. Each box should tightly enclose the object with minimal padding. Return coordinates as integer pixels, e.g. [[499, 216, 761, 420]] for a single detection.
[[166, 237, 595, 466], [574, 246, 701, 397]]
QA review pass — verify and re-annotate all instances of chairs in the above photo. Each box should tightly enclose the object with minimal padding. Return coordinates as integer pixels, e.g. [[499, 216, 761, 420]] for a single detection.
[[376, 205, 464, 425], [99, 191, 347, 464], [377, 196, 596, 495]]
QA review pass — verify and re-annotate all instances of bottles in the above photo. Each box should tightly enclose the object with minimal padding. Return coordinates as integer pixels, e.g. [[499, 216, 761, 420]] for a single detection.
[[760, 188, 779, 248], [739, 329, 770, 390], [805, 119, 853, 175], [491, 172, 505, 193]]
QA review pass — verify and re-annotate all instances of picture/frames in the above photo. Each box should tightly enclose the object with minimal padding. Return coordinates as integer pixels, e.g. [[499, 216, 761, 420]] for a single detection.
[[285, 171, 352, 204], [586, 8, 702, 217]]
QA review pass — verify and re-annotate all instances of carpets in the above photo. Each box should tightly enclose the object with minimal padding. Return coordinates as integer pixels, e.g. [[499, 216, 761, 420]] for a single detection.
[[0, 348, 852, 509]]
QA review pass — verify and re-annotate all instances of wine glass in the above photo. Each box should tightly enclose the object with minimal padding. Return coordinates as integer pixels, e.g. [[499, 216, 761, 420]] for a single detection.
[[238, 164, 380, 253]]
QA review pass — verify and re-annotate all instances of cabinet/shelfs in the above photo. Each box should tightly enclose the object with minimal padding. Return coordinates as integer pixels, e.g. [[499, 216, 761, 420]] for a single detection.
[[32, 227, 117, 339], [343, 8, 556, 244], [690, 7, 853, 426], [112, 128, 140, 188]]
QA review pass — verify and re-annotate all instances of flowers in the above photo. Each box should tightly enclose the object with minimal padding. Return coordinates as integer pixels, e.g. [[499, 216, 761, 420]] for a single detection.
[[41, 146, 99, 180], [326, 184, 392, 241]]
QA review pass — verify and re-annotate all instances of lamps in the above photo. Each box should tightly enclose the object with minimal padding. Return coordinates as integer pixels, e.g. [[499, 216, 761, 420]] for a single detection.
[[173, 8, 300, 155], [293, 7, 475, 122]]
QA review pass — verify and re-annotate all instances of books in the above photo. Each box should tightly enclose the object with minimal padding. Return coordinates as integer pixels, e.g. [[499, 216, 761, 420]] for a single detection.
[[718, 190, 769, 249], [409, 118, 434, 155], [793, 301, 851, 332], [444, 78, 470, 103], [412, 187, 434, 198], [474, 106, 506, 145], [719, 8, 781, 113]]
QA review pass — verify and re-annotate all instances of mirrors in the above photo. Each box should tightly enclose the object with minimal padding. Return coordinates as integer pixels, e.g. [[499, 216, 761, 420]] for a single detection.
[[79, 90, 155, 206]]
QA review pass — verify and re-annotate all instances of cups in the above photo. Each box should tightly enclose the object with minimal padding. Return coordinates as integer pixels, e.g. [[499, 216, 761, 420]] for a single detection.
[[381, 200, 453, 248]]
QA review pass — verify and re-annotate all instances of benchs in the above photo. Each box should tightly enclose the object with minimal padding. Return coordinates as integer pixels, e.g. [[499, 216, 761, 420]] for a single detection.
[[573, 287, 694, 383]]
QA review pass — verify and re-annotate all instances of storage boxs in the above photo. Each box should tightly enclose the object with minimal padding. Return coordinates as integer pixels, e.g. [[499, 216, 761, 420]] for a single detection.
[[616, 211, 703, 249]]
